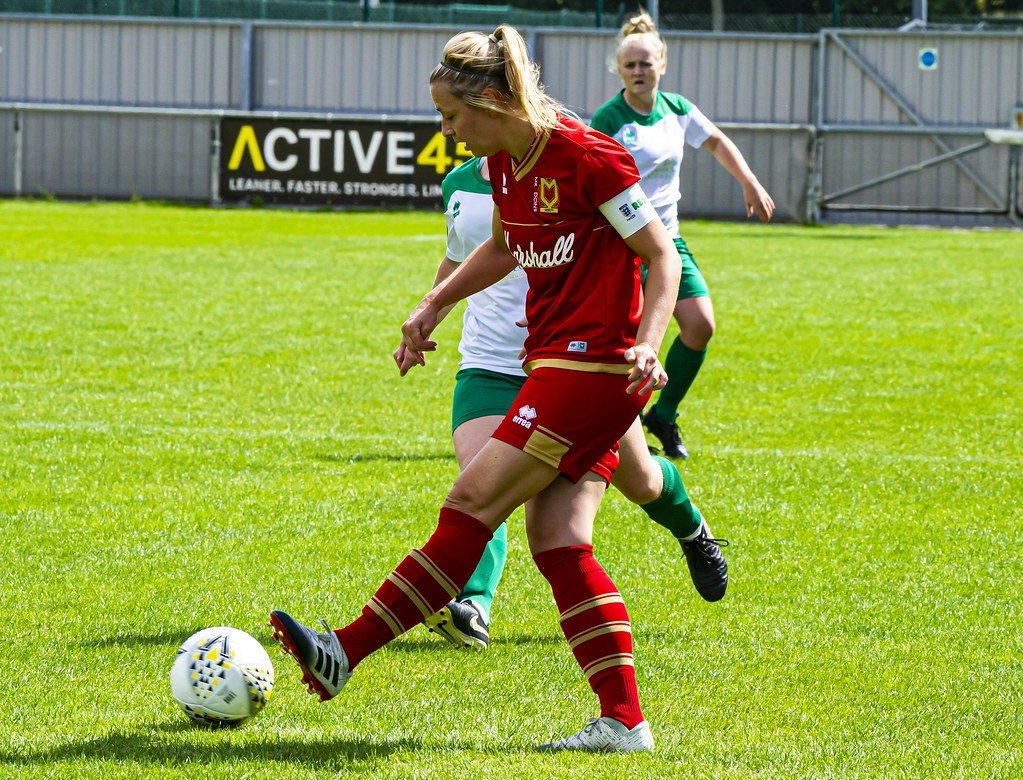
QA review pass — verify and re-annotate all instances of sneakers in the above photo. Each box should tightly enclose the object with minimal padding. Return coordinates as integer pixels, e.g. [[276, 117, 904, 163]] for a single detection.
[[421, 600, 489, 651], [678, 505, 730, 602], [270, 610, 354, 702], [643, 404, 687, 458], [533, 716, 656, 755]]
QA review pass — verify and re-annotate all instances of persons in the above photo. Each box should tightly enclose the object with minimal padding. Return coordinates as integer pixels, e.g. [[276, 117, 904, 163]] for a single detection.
[[394, 156, 730, 653], [587, 11, 776, 461], [264, 21, 684, 756]]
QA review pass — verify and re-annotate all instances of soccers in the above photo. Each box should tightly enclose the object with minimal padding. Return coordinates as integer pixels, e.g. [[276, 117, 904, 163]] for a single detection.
[[170, 625, 274, 727]]
